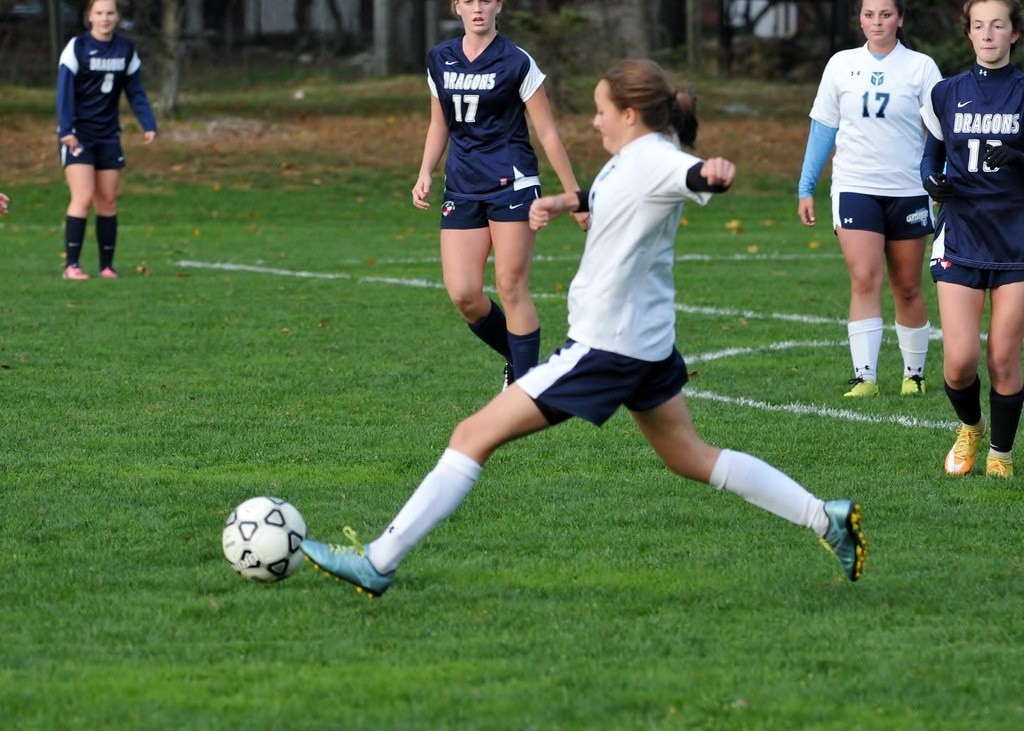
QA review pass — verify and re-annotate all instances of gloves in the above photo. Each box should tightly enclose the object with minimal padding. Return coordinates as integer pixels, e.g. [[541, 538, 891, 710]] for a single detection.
[[925, 173, 946, 203], [982, 143, 1017, 170]]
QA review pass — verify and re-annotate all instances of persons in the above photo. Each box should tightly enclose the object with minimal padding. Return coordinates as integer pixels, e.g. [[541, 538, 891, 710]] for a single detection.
[[798, 0, 942, 398], [411, 0, 591, 394], [297, 59, 866, 600], [919, 0, 1024, 478], [56, 0, 157, 280]]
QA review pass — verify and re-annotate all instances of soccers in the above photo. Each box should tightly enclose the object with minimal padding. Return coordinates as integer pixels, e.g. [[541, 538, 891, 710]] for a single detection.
[[220, 495, 309, 585]]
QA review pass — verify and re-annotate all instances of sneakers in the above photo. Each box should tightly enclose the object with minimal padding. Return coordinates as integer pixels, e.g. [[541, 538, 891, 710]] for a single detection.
[[100, 266, 119, 279], [844, 377, 880, 399], [502, 362, 514, 393], [900, 373, 926, 397], [945, 412, 987, 474], [62, 262, 88, 280], [818, 500, 868, 582], [299, 526, 398, 600], [985, 452, 1014, 481]]
